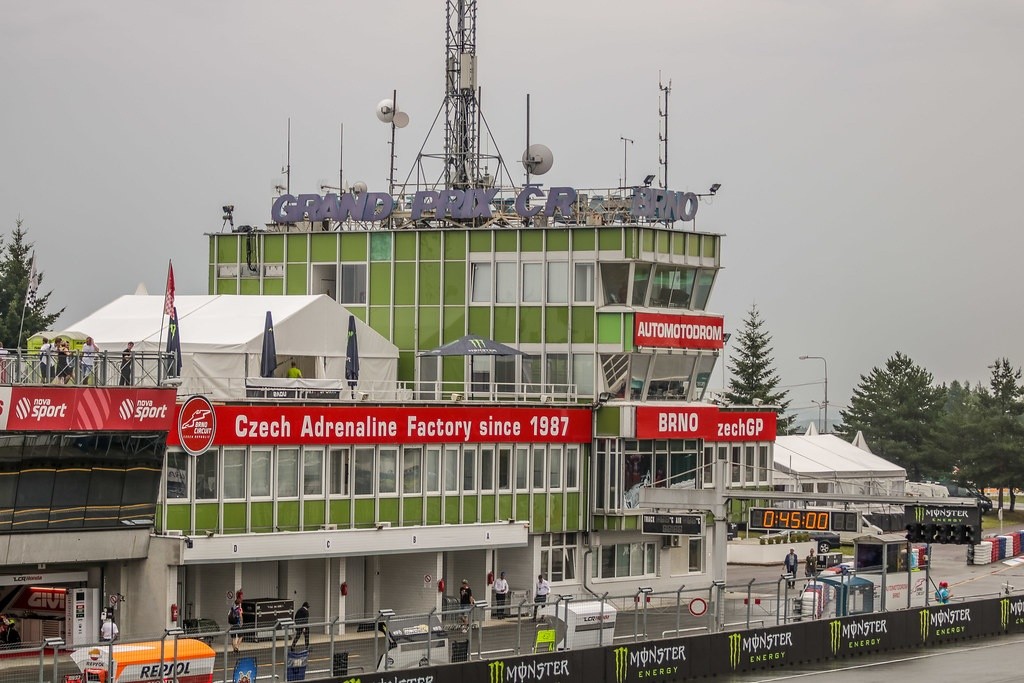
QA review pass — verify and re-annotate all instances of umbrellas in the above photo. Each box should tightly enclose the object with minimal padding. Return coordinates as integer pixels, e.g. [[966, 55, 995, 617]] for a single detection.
[[166, 307, 182, 378], [260, 311, 277, 377], [416, 336, 528, 399], [345, 316, 359, 399]]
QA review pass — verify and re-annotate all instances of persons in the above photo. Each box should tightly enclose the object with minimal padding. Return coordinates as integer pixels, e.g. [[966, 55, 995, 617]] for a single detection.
[[2, 624, 21, 649], [494, 572, 509, 620], [935, 581, 953, 604], [805, 548, 817, 582], [228, 599, 244, 653], [119, 342, 135, 385], [459, 579, 473, 626], [291, 601, 313, 652], [782, 547, 798, 588], [81, 337, 100, 385], [0, 338, 74, 385], [100, 616, 118, 642], [286, 361, 303, 378], [529, 575, 551, 622]]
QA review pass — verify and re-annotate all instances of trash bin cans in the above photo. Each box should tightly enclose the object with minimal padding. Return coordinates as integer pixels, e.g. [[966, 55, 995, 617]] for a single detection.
[[184, 619, 199, 634], [197, 618, 219, 643]]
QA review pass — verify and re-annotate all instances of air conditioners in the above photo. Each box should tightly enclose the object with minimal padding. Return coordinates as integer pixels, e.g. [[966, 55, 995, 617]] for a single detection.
[[665, 535, 682, 547]]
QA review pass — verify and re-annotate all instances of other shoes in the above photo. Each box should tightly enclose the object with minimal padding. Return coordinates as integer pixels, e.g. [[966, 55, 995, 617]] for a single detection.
[[462, 629, 467, 633], [232, 648, 240, 654]]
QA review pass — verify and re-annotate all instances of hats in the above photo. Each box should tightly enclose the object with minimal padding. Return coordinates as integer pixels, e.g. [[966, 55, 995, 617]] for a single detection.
[[462, 579, 468, 584], [790, 547, 794, 550], [810, 547, 814, 551], [303, 602, 310, 608]]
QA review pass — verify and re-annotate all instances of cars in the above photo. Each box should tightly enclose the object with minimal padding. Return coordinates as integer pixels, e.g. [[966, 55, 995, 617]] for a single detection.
[[833, 516, 883, 545], [809, 531, 841, 553], [931, 481, 993, 515]]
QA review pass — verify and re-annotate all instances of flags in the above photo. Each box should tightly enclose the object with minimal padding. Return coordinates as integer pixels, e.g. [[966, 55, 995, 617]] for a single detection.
[[164, 261, 175, 320]]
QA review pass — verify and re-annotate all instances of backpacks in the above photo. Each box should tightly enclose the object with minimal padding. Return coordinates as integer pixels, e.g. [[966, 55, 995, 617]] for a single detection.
[[228, 606, 240, 624]]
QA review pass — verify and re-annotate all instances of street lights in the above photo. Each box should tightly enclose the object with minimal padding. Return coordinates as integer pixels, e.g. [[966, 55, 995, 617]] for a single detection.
[[812, 400, 829, 434], [799, 356, 827, 434]]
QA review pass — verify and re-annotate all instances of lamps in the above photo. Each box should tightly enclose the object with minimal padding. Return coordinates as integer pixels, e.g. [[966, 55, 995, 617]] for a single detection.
[[594, 392, 610, 410], [377, 523, 383, 530], [507, 518, 515, 524], [185, 534, 193, 549], [204, 530, 214, 537], [696, 183, 721, 197], [643, 174, 656, 187]]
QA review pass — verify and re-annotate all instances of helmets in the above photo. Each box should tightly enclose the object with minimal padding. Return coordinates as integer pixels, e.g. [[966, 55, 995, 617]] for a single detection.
[[940, 581, 948, 587]]
